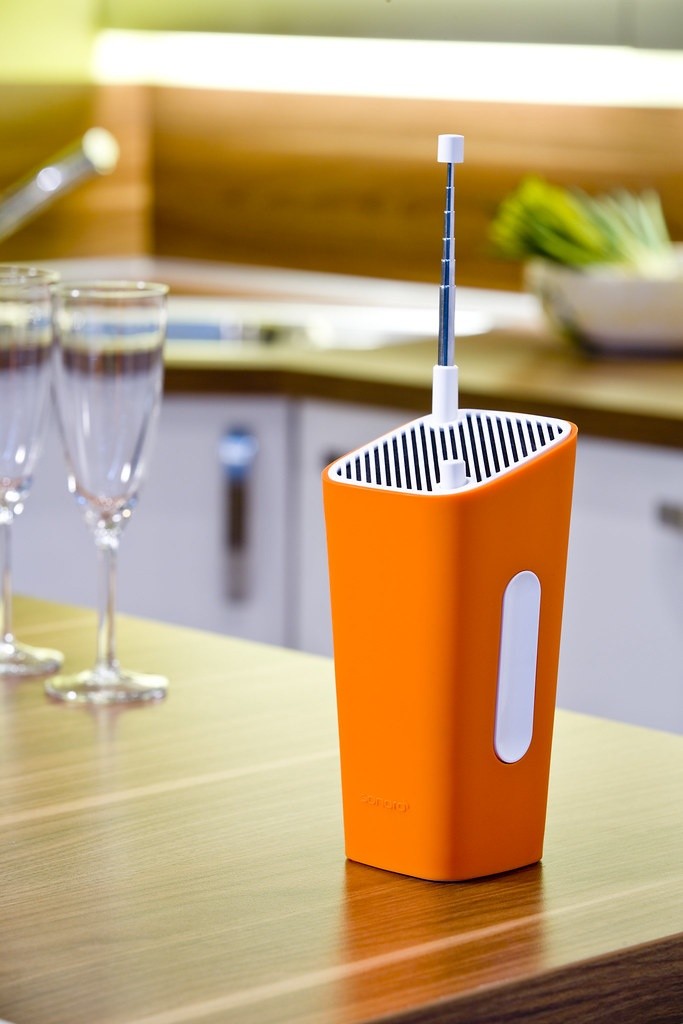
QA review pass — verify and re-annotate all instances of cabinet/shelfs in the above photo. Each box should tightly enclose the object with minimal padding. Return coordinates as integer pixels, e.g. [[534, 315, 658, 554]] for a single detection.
[[0, 360, 293, 646], [297, 368, 680, 738]]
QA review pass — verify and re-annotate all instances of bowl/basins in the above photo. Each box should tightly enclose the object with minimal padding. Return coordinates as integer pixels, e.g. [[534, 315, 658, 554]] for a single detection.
[[529, 246, 683, 355]]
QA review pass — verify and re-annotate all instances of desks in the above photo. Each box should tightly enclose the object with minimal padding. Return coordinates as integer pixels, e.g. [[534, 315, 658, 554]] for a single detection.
[[3, 589, 681, 1023]]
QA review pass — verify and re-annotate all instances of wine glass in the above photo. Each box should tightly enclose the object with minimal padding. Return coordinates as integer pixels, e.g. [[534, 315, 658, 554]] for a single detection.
[[41, 279, 174, 702], [0, 262, 65, 677]]
